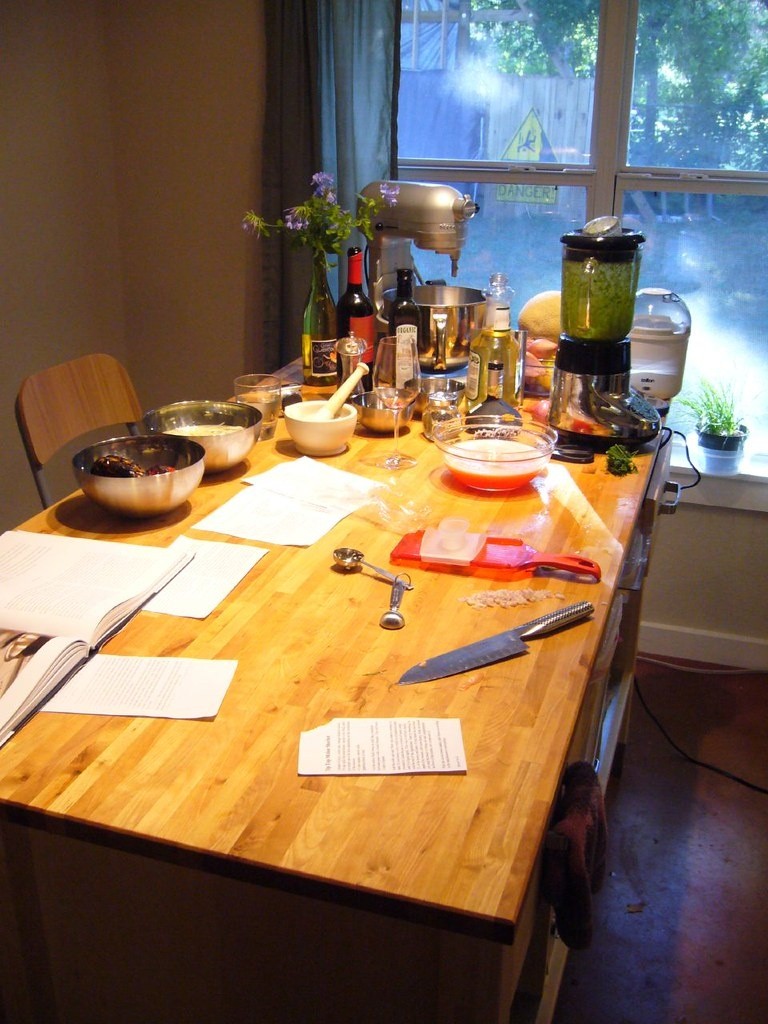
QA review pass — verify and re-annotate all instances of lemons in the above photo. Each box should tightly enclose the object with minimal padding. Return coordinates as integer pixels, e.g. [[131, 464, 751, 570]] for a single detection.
[[539, 357, 554, 391]]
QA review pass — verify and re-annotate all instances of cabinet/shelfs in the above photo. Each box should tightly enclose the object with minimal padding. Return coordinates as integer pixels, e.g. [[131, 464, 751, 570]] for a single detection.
[[0, 357, 682, 1024]]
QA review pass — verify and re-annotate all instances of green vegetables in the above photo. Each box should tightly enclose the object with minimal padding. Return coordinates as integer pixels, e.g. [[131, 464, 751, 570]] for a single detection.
[[606, 444, 639, 476]]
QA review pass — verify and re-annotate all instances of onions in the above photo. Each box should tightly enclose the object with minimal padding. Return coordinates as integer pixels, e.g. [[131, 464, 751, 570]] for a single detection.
[[522, 399, 550, 424]]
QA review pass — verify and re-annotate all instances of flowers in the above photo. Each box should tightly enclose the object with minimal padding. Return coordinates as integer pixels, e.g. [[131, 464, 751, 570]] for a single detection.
[[240, 172, 400, 301]]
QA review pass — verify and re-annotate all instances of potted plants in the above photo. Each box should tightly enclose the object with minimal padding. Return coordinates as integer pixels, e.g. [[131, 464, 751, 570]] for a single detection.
[[669, 381, 749, 476]]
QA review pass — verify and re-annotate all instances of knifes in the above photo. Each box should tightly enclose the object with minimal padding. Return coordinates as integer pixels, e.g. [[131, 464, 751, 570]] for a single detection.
[[397, 600, 595, 686]]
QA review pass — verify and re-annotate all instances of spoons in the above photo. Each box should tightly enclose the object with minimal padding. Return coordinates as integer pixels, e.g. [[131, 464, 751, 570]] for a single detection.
[[380, 582, 405, 630], [333, 548, 414, 590]]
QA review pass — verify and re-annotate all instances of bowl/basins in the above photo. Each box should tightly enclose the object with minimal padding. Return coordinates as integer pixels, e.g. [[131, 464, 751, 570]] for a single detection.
[[383, 285, 489, 372], [431, 414, 558, 493], [143, 400, 263, 475], [71, 433, 206, 520], [404, 377, 466, 415], [283, 400, 357, 457], [350, 390, 416, 432]]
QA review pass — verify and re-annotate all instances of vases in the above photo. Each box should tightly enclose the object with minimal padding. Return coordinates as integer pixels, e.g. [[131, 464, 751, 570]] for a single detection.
[[301, 245, 337, 386]]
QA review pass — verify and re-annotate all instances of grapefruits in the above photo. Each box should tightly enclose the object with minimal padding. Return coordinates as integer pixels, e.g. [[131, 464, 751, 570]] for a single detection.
[[517, 290, 561, 342]]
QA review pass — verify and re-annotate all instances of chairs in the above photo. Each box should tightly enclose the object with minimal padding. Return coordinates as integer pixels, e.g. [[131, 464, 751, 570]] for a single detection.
[[14, 352, 144, 513]]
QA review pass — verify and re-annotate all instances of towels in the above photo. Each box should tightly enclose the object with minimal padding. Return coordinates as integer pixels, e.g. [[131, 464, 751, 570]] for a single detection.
[[542, 760, 608, 953]]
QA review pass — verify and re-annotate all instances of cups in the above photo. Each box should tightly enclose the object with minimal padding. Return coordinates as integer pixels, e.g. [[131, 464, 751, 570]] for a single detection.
[[233, 373, 281, 442]]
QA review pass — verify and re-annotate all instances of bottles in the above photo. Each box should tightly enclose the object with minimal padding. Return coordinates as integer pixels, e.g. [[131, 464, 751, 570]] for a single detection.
[[465, 272, 524, 410], [389, 269, 423, 390], [336, 247, 377, 407]]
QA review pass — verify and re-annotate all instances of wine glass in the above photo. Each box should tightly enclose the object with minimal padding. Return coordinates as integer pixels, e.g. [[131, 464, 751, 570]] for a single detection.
[[371, 336, 422, 471]]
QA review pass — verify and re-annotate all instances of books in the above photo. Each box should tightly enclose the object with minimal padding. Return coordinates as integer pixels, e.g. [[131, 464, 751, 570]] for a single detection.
[[0, 530, 195, 749]]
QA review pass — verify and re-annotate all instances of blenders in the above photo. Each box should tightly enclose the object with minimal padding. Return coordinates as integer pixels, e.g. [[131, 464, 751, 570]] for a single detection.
[[542, 216, 663, 465], [353, 180, 489, 393], [626, 287, 692, 417]]
